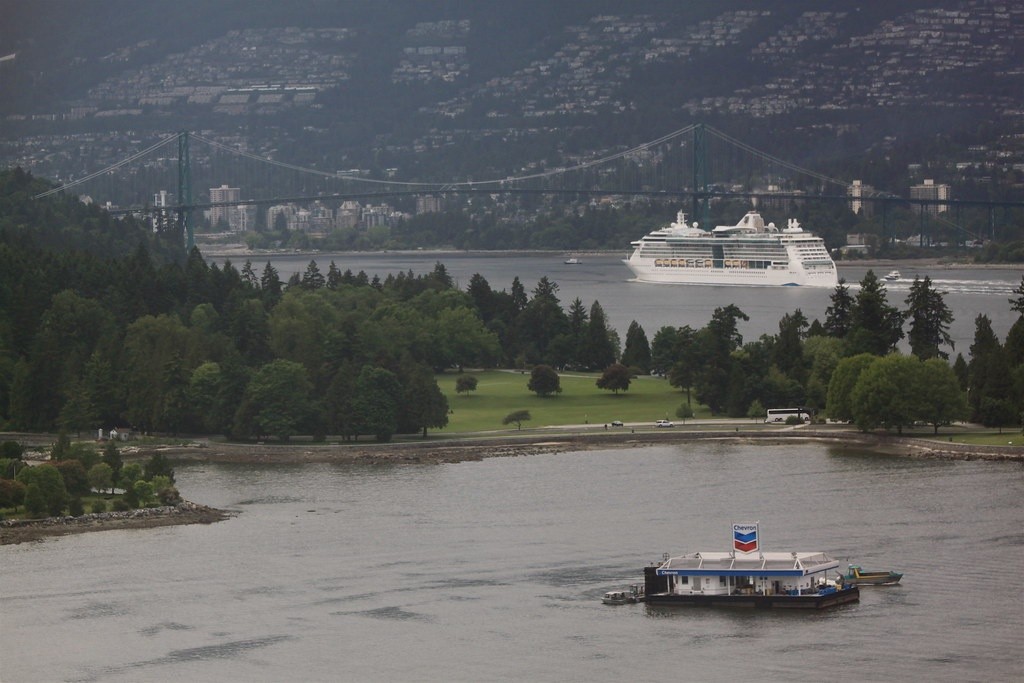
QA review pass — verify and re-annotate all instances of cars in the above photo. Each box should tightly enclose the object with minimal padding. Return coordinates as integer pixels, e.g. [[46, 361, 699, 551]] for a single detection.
[[611, 421, 623, 426]]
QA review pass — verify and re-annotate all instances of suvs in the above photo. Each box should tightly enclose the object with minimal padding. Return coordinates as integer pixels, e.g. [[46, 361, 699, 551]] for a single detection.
[[656, 420, 675, 428]]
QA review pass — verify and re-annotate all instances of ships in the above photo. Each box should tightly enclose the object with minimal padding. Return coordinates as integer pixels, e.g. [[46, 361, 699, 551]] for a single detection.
[[621, 204, 838, 287]]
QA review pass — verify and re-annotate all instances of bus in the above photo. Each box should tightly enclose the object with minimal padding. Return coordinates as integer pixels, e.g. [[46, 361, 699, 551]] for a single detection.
[[767, 408, 810, 422]]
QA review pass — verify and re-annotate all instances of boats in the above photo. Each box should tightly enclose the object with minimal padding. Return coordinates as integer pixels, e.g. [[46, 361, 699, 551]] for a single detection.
[[884, 270, 901, 281], [564, 258, 582, 264], [600, 584, 646, 605], [836, 564, 903, 586], [655, 259, 739, 266], [642, 546, 860, 609]]
